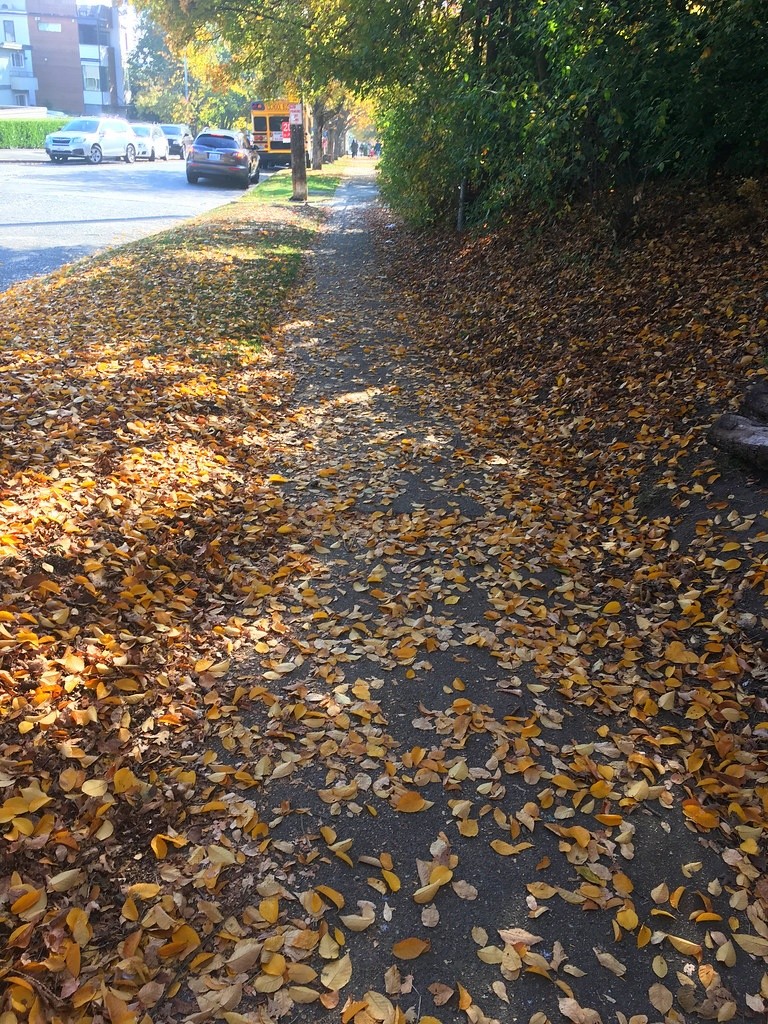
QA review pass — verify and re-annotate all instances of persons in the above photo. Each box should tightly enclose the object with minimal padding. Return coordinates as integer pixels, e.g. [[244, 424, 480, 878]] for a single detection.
[[350, 140, 382, 159]]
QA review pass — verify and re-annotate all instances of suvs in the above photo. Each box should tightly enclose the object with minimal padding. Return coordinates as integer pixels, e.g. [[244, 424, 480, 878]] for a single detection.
[[160, 125, 193, 160], [186, 127, 260, 188], [131, 123, 169, 162], [44, 117, 139, 163]]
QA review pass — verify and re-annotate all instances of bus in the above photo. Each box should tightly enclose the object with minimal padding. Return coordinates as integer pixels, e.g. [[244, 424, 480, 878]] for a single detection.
[[250, 101, 312, 170]]
